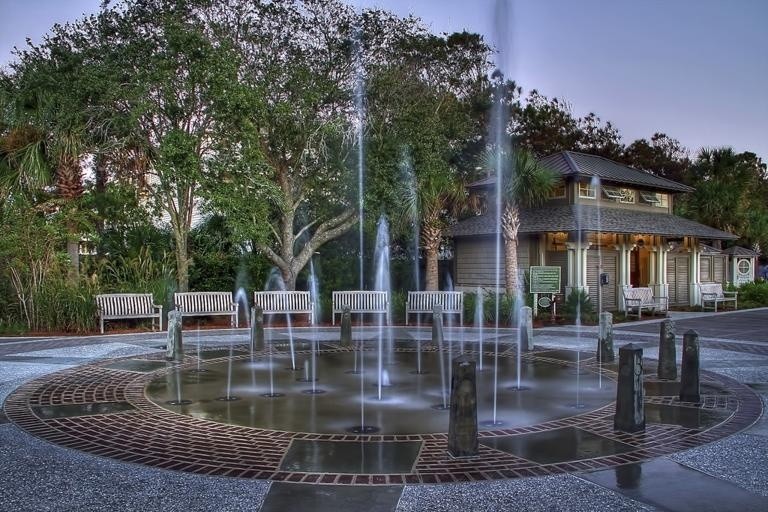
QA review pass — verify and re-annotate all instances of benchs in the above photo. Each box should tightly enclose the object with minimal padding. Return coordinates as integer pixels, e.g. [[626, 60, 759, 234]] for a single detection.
[[174, 292, 240, 330], [253, 291, 315, 326], [699, 283, 739, 312], [621, 287, 670, 319], [96, 292, 164, 335], [331, 290, 390, 326], [405, 291, 465, 327]]
